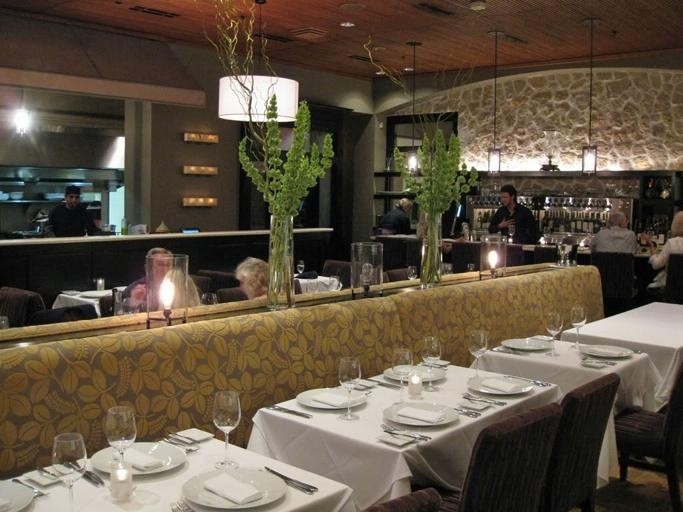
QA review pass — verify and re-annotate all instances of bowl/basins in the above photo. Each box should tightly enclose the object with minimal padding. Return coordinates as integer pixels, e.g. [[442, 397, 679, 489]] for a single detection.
[[44, 192, 67, 201], [0, 192, 24, 200]]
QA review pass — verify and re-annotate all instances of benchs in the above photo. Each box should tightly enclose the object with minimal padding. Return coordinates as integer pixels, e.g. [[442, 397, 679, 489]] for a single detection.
[[1, 274, 408, 352], [2, 291, 406, 478]]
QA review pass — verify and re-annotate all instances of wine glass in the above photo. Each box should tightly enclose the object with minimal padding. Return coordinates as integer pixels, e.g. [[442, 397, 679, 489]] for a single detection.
[[337, 355, 362, 421], [545, 310, 563, 359], [391, 347, 414, 397], [51, 432, 88, 511], [213, 389, 242, 472], [104, 406, 139, 463], [420, 335, 442, 394], [570, 305, 587, 354], [466, 328, 488, 376]]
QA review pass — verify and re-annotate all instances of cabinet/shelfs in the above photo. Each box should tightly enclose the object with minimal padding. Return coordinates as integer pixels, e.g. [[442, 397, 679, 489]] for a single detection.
[[465, 194, 537, 232], [374, 167, 420, 224], [538, 196, 631, 234]]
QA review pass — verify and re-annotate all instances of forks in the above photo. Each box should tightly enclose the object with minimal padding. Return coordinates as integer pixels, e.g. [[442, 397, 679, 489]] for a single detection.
[[169, 500, 193, 512], [10, 478, 51, 498]]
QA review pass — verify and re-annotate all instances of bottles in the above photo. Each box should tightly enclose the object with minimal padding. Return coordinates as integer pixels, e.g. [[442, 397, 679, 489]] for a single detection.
[[478, 209, 609, 234], [99, 218, 128, 234], [637, 218, 666, 246]]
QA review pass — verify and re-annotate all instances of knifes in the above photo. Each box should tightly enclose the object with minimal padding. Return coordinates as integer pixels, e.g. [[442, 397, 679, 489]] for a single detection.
[[263, 466, 319, 494], [62, 454, 105, 488]]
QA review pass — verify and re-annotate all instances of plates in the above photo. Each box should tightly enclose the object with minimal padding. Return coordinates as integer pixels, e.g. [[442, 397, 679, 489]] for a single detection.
[[0, 480, 35, 511], [466, 374, 531, 396], [578, 343, 633, 359], [297, 387, 365, 410], [382, 402, 460, 425], [383, 364, 444, 382], [91, 440, 186, 475], [499, 337, 552, 352], [180, 468, 287, 509]]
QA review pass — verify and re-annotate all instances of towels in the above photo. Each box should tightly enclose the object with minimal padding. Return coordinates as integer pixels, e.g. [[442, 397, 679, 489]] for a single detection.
[[202, 470, 261, 504], [481, 376, 522, 394], [312, 391, 351, 406], [112, 447, 162, 470], [408, 367, 435, 379], [396, 405, 443, 422]]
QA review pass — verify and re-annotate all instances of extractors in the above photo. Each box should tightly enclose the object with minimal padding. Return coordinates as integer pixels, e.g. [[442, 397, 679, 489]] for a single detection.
[[0, 165, 123, 195]]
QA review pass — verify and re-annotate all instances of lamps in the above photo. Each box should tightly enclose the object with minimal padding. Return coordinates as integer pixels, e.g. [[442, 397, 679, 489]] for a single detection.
[[485, 30, 505, 176], [346, 241, 385, 299], [179, 196, 218, 207], [215, 1, 301, 124], [183, 132, 219, 143], [403, 41, 420, 170], [539, 127, 562, 170], [141, 253, 191, 328], [13, 89, 31, 135], [579, 1, 603, 175], [182, 165, 218, 176]]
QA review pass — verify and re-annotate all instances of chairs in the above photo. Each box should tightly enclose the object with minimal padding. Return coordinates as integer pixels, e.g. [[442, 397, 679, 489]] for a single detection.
[[376, 235, 605, 364], [185, 256, 419, 275], [589, 247, 636, 314], [652, 253, 682, 306], [558, 372, 622, 511], [362, 487, 446, 511], [614, 357, 681, 511], [1, 285, 96, 332], [411, 402, 562, 510], [96, 277, 149, 315]]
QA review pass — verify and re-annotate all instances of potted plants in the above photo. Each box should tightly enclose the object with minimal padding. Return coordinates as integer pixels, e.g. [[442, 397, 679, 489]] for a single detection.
[[198, 1, 336, 310], [355, 34, 478, 285]]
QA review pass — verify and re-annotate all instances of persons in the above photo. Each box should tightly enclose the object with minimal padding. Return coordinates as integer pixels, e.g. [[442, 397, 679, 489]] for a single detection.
[[122, 247, 269, 313], [48, 185, 96, 237], [489, 185, 538, 243], [378, 197, 414, 235], [646, 211, 683, 288], [417, 212, 452, 256], [590, 211, 642, 298]]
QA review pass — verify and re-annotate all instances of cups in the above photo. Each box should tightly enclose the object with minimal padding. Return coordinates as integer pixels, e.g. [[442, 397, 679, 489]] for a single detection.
[[407, 265, 417, 280], [93, 278, 219, 318], [538, 231, 593, 248], [469, 228, 514, 245], [296, 259, 305, 274], [0, 315, 8, 330]]
